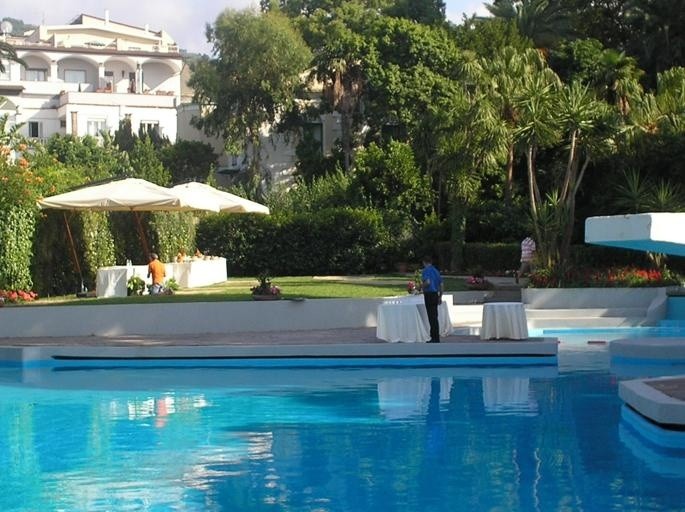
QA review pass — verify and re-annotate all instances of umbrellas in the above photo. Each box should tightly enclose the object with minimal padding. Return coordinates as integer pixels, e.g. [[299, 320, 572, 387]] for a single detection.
[[131, 179, 271, 263], [35, 174, 184, 281]]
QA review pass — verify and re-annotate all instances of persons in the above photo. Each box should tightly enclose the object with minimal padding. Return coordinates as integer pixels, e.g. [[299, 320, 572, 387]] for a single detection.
[[414, 255, 447, 344], [514, 230, 537, 284], [145, 253, 166, 286]]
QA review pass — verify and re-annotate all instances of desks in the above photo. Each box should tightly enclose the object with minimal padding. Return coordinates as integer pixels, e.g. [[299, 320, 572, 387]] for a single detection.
[[479, 300, 530, 342], [161, 257, 227, 290], [93, 261, 153, 301], [375, 293, 455, 345]]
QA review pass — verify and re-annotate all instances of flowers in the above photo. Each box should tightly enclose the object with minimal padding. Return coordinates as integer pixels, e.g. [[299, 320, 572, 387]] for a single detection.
[[248, 268, 283, 296]]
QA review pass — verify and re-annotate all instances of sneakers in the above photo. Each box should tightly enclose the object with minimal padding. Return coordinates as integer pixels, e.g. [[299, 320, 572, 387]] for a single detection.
[[426, 339, 441, 343], [515, 272, 520, 283]]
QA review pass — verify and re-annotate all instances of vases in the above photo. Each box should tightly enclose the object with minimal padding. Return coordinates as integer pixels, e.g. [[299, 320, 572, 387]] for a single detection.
[[252, 295, 280, 300]]
